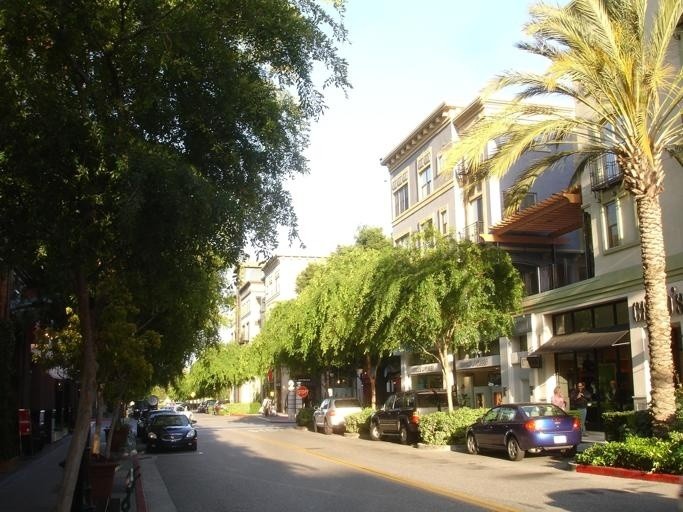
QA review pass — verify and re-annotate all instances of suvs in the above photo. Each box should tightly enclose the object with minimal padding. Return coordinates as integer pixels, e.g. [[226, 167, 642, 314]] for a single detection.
[[369, 388, 459, 446], [312, 397, 364, 434]]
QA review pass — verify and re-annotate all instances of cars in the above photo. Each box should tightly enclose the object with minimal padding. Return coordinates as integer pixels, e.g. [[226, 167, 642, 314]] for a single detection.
[[197, 399, 230, 414], [133, 399, 198, 453], [465, 402, 582, 461]]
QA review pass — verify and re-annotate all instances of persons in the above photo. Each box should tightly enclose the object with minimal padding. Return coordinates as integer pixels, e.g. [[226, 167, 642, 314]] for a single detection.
[[569, 381, 591, 437], [608, 379, 618, 400], [549, 384, 565, 411]]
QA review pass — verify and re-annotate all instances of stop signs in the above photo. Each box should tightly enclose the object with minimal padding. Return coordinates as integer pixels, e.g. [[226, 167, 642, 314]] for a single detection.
[[296, 385, 309, 399]]
